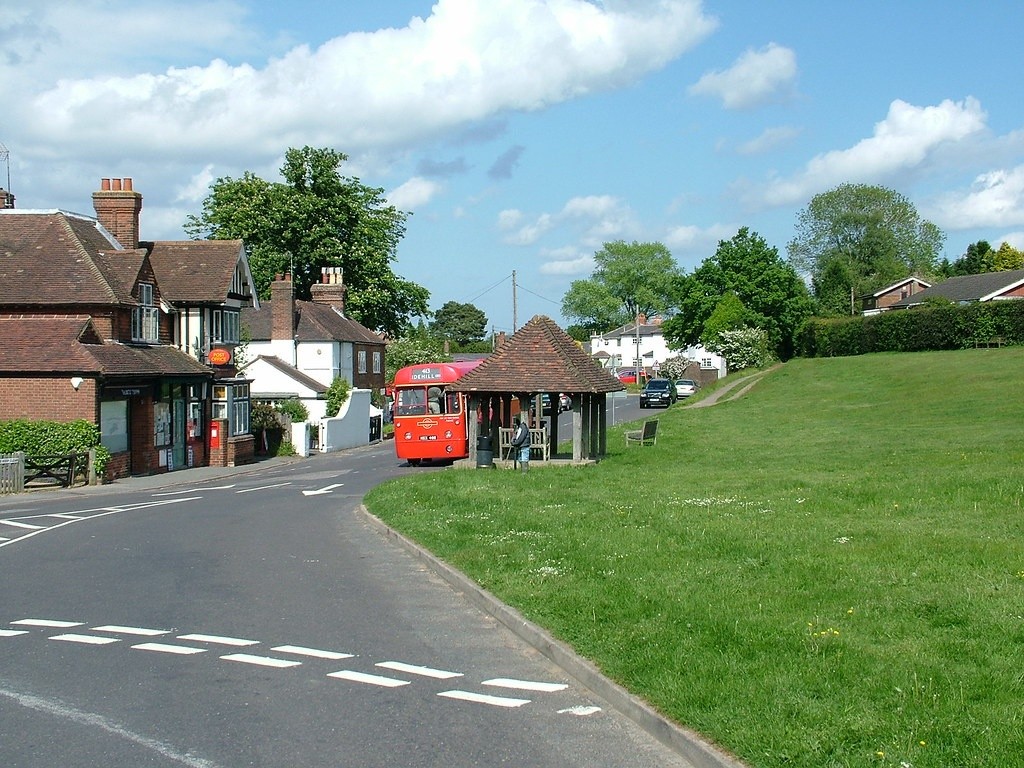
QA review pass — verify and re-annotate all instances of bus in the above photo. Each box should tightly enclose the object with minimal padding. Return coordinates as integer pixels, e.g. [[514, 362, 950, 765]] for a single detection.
[[386, 356, 488, 465]]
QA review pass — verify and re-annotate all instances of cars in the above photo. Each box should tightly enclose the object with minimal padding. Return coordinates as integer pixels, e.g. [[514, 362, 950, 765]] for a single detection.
[[530, 394, 563, 416], [617, 371, 637, 384], [559, 393, 573, 411], [674, 379, 702, 399]]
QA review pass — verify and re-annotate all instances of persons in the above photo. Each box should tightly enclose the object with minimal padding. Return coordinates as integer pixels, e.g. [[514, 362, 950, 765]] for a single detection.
[[511, 419, 530, 475]]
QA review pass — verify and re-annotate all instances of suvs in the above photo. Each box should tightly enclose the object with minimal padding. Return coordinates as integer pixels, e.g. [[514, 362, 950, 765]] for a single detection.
[[639, 378, 678, 410]]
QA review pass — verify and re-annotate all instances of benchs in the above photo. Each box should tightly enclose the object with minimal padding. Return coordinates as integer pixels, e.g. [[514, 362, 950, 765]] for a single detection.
[[624, 418, 659, 446], [496, 426, 551, 463]]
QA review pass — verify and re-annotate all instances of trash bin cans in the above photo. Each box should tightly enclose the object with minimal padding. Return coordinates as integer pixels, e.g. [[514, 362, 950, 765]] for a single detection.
[[477, 436, 494, 468]]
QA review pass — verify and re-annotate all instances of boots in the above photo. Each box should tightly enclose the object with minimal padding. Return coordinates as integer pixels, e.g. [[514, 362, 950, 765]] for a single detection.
[[521, 461, 528, 475]]
